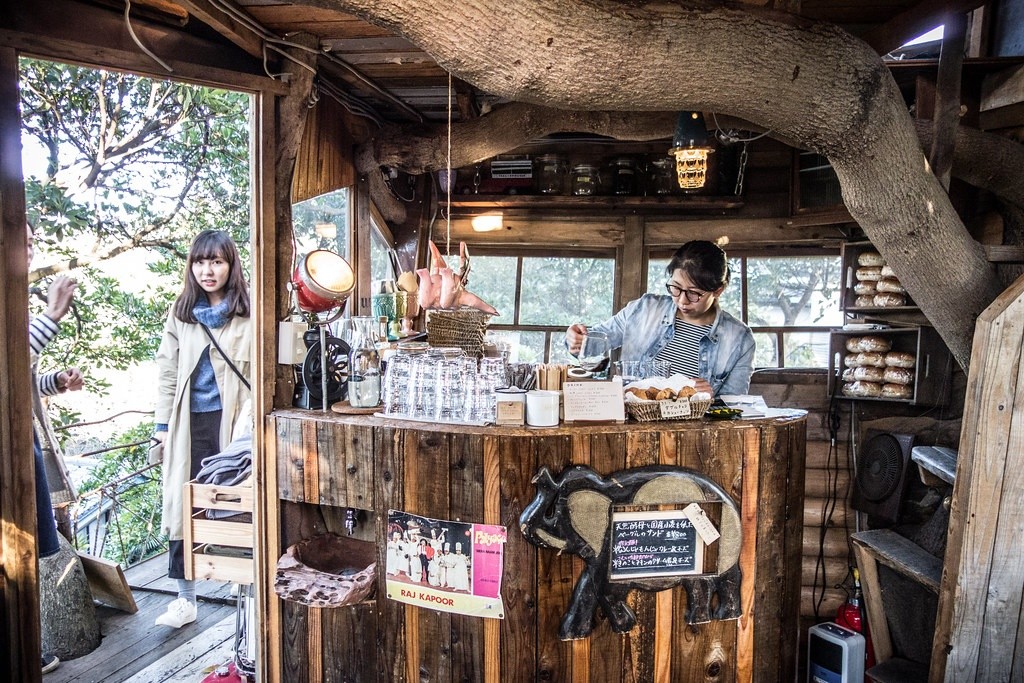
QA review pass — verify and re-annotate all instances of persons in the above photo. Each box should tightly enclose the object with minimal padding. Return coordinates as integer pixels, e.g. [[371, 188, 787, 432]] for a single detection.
[[387, 530, 471, 593], [150, 230, 251, 629], [27, 221, 85, 674], [564, 240, 756, 397]]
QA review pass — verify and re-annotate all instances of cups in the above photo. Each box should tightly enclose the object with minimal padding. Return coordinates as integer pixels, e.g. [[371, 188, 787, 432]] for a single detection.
[[614, 360, 671, 379], [383, 343, 559, 426]]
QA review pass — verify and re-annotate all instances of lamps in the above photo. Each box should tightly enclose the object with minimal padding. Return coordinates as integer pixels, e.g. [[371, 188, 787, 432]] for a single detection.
[[286, 248, 357, 414], [668, 112, 715, 194], [234, 582, 255, 678]]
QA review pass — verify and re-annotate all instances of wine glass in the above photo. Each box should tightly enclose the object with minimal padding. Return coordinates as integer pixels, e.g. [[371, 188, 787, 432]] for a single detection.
[[375, 292, 421, 340]]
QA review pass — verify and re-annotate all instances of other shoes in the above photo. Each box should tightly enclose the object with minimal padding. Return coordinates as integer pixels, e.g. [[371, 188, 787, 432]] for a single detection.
[[41, 655, 59, 675]]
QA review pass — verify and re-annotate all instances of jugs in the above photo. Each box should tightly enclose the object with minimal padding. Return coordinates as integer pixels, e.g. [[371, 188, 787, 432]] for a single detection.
[[578, 331, 610, 372]]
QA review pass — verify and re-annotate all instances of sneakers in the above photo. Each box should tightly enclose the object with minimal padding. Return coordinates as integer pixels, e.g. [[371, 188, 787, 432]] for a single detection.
[[230, 583, 245, 596], [155, 598, 197, 628]]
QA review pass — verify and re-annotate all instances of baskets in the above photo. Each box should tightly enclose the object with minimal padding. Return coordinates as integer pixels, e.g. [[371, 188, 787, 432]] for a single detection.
[[625, 399, 714, 423], [426, 309, 492, 365]]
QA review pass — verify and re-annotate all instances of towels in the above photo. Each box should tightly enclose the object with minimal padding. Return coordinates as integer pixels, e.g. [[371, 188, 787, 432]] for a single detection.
[[194, 432, 254, 560]]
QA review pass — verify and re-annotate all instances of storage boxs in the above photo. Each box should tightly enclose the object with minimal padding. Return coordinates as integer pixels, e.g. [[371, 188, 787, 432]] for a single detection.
[[183, 477, 256, 587], [826, 239, 955, 408]]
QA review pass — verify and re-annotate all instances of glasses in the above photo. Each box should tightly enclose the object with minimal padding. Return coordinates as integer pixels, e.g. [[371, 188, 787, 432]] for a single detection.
[[665, 274, 720, 303]]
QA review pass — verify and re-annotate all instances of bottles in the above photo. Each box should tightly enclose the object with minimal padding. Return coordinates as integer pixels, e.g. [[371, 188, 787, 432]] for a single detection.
[[534, 154, 677, 198], [349, 316, 379, 407]]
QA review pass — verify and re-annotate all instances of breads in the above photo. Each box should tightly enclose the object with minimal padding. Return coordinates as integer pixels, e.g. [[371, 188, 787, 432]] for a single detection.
[[625, 386, 697, 400], [841, 336, 916, 399], [854, 252, 909, 307]]
